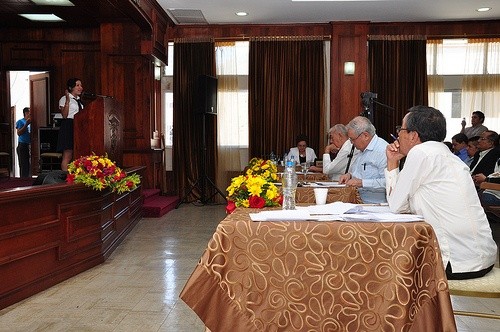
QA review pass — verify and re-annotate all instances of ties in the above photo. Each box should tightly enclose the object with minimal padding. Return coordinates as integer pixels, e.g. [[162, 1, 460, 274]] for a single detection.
[[74, 98, 82, 111]]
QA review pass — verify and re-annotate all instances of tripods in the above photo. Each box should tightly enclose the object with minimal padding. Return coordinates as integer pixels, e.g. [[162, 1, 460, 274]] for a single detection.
[[176, 116, 229, 207]]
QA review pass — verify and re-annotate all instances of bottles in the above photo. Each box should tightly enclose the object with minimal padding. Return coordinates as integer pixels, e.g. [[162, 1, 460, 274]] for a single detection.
[[283, 153, 288, 164], [281, 162, 298, 210], [270, 152, 274, 162], [290, 156, 296, 172]]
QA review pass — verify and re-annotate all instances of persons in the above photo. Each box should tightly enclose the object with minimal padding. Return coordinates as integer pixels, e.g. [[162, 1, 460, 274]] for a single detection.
[[384, 105, 497, 280], [59, 78, 84, 171], [339, 115, 389, 202], [323, 124, 361, 174], [16, 107, 31, 177], [452, 130, 500, 206], [285, 135, 317, 165], [461, 111, 488, 138]]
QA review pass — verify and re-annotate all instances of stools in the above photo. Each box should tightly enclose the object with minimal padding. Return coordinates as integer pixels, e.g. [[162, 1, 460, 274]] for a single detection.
[[40, 153, 63, 172]]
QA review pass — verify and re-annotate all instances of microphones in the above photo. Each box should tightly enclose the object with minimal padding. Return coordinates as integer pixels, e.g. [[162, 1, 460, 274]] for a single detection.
[[82, 92, 95, 97]]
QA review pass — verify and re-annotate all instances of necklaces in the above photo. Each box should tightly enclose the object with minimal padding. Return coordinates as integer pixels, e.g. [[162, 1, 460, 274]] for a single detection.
[[300, 152, 305, 157]]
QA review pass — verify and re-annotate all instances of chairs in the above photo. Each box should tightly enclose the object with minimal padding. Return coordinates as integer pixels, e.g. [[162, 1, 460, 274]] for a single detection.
[[447, 267, 500, 320]]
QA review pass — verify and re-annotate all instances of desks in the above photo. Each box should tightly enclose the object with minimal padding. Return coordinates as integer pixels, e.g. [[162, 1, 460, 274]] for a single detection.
[[178, 164, 458, 332]]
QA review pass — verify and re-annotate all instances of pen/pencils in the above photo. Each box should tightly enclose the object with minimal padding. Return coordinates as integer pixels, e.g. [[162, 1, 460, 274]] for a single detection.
[[390, 134, 397, 140]]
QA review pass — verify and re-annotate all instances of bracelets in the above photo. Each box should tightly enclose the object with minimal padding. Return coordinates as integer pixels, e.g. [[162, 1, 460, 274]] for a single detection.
[[65, 105, 68, 106]]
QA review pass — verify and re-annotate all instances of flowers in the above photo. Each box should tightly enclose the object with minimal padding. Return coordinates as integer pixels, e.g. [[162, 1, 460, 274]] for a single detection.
[[66, 151, 140, 194], [226, 158, 283, 214]]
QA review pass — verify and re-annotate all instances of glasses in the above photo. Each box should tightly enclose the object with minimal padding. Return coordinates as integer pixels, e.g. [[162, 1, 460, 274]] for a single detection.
[[479, 136, 487, 140], [396, 126, 406, 132], [348, 130, 364, 142]]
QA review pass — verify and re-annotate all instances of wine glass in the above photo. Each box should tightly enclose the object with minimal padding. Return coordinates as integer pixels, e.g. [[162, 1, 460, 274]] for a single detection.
[[300, 162, 310, 185]]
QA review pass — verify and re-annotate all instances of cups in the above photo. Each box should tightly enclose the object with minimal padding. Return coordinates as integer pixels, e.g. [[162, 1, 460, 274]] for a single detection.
[[274, 155, 280, 161], [313, 188, 328, 205]]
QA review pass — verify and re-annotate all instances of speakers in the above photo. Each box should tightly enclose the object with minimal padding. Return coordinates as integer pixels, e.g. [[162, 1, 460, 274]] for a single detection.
[[39, 127, 63, 171], [34, 171, 67, 186], [195, 74, 217, 115]]
